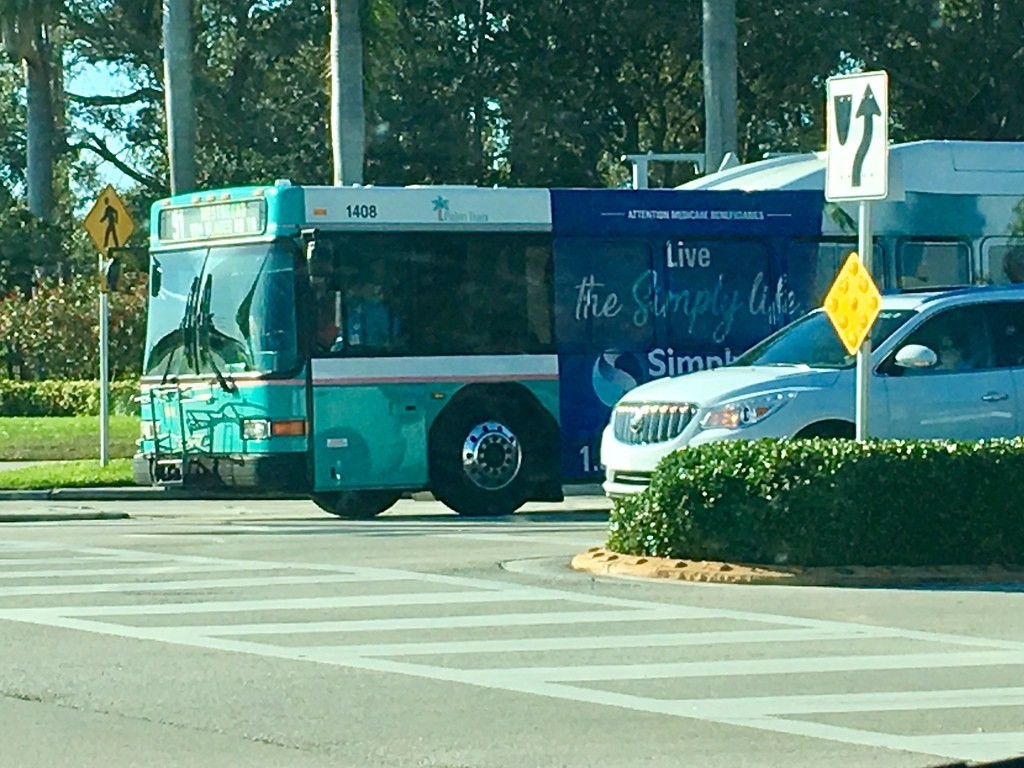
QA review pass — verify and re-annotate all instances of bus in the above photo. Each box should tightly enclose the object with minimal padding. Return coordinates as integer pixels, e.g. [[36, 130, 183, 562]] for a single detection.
[[139, 139, 1024, 519]]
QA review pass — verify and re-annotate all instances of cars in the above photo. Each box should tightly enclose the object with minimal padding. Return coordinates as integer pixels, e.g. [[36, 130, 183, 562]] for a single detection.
[[602, 290, 1021, 519]]
